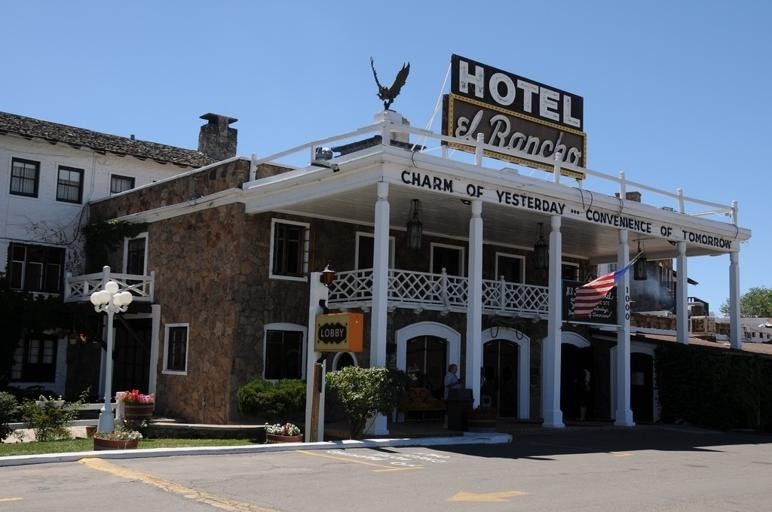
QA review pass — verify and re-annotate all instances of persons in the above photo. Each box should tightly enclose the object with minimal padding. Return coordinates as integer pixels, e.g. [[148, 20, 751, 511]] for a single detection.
[[443, 362, 463, 429]]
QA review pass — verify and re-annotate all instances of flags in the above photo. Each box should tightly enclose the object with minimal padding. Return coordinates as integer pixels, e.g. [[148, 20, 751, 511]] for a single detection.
[[573, 255, 639, 319]]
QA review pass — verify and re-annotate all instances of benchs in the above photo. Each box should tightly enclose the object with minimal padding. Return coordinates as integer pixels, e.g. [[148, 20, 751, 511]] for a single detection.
[[406, 387, 448, 425]]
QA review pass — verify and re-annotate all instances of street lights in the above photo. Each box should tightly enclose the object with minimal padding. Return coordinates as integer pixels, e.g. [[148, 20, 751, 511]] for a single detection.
[[90, 281, 133, 433]]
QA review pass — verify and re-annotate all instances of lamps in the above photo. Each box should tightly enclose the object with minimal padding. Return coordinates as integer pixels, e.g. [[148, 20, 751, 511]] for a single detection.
[[535, 222, 549, 270], [634, 240, 648, 280], [406, 199, 422, 249], [320, 269, 335, 288]]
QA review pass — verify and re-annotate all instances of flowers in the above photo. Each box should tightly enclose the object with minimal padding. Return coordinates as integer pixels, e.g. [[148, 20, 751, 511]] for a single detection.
[[117, 431, 142, 441], [265, 422, 301, 437], [115, 390, 154, 405]]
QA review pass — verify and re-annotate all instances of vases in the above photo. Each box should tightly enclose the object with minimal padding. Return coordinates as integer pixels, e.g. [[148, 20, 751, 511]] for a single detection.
[[94, 433, 137, 451], [266, 434, 302, 444], [125, 403, 153, 425]]
